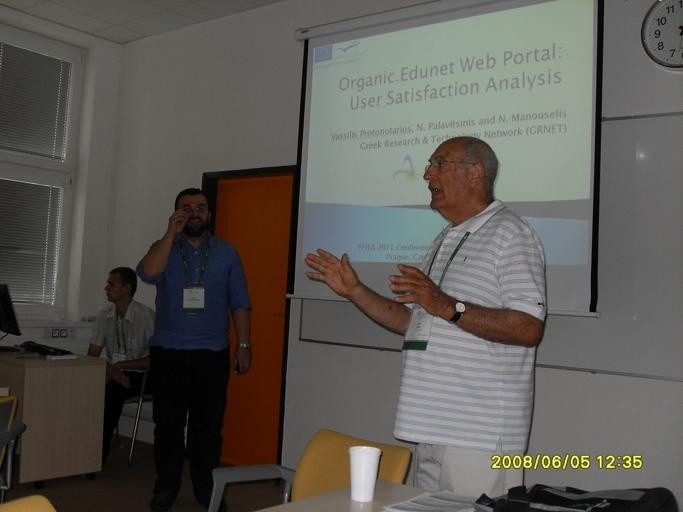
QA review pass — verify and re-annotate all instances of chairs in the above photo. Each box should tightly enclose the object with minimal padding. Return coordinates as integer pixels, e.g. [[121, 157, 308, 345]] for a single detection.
[[209, 428, 412, 511], [1, 395, 27, 490], [119, 366, 191, 467]]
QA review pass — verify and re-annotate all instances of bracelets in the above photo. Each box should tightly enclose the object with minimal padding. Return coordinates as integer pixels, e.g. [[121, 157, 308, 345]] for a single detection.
[[238, 341, 250, 349]]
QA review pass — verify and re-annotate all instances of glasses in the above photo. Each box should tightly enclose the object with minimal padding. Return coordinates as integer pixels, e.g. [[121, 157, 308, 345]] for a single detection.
[[424, 159, 474, 174], [177, 207, 211, 213]]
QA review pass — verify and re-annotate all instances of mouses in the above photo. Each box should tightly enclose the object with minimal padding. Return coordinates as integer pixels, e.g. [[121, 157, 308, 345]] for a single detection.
[[20, 341, 36, 346]]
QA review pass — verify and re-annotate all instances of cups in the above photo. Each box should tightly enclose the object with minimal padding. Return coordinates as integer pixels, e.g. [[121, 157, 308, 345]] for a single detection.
[[347, 445, 381, 503]]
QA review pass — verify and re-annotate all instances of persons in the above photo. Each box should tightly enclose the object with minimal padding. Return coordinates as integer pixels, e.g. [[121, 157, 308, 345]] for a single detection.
[[305, 137, 547, 500], [89, 267, 155, 468], [136, 187, 253, 512]]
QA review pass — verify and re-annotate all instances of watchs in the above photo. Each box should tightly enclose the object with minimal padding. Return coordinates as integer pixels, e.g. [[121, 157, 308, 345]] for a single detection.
[[449, 300, 465, 323]]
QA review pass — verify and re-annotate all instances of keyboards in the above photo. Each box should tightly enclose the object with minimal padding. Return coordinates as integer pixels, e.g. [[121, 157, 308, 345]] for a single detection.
[[24, 344, 77, 360]]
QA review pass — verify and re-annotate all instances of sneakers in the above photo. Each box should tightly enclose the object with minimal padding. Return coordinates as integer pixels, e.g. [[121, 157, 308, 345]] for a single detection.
[[149, 485, 178, 511], [194, 490, 228, 511]]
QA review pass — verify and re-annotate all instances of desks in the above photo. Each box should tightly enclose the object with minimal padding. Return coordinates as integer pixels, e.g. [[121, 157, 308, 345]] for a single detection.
[[257, 480, 494, 511], [0, 345, 107, 485]]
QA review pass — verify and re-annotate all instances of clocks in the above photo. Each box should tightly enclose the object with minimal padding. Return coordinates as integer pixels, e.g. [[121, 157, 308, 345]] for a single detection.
[[640, 0, 683, 69]]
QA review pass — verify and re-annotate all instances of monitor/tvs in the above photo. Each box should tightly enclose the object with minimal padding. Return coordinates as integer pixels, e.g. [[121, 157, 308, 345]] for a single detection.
[[0, 283, 21, 353]]
